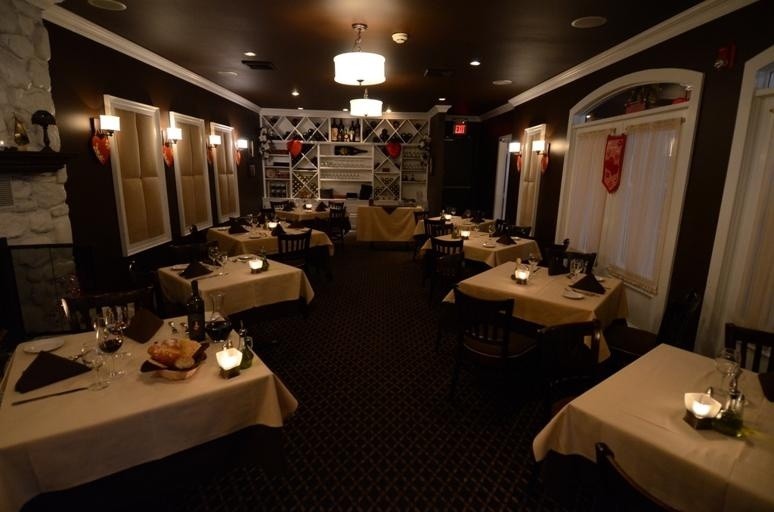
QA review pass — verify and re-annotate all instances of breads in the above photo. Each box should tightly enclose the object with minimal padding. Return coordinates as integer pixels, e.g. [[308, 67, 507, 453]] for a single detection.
[[148, 338, 202, 368]]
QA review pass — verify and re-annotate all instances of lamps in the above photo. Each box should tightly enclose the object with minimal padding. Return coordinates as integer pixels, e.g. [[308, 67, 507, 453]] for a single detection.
[[91, 114, 121, 164], [162, 127, 183, 168], [332, 23, 386, 86], [509, 142, 522, 172], [532, 140, 549, 173], [207, 135, 221, 165], [234, 139, 248, 165], [349, 86, 383, 118]]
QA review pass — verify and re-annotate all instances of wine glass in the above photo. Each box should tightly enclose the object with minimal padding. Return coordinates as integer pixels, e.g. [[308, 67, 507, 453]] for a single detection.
[[570, 259, 584, 284], [208, 247, 219, 270], [247, 214, 265, 238], [217, 256, 229, 275], [713, 348, 741, 396], [488, 224, 496, 241], [81, 314, 131, 393], [529, 254, 539, 268]]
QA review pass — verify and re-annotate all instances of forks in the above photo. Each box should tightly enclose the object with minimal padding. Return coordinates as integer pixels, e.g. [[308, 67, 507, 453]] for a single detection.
[[168, 321, 190, 337]]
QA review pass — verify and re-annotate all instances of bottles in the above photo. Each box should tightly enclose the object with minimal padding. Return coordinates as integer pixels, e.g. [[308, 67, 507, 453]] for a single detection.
[[237, 329, 253, 369], [334, 146, 368, 155], [185, 280, 205, 342], [268, 118, 422, 143], [209, 292, 229, 321]]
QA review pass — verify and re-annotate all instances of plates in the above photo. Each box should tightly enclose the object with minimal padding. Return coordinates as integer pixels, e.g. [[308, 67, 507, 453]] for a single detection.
[[24, 339, 64, 354], [482, 243, 497, 248], [511, 236, 521, 240], [561, 292, 584, 299], [172, 264, 189, 270], [594, 275, 605, 281]]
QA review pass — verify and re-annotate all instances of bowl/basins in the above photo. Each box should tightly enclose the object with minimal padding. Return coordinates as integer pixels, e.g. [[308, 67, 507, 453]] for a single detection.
[[204, 321, 232, 342]]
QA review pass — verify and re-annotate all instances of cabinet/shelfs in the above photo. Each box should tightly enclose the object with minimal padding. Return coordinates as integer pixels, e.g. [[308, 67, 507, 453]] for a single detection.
[[259, 110, 431, 216]]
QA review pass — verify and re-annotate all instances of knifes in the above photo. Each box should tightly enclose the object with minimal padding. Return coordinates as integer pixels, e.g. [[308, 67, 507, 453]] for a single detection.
[[12, 387, 90, 406]]
